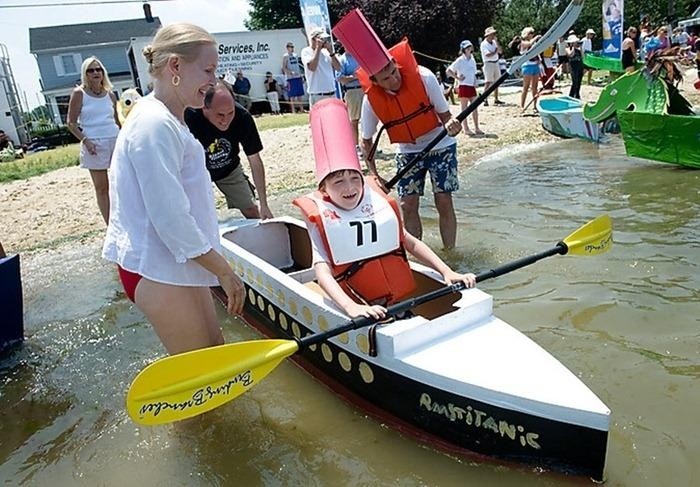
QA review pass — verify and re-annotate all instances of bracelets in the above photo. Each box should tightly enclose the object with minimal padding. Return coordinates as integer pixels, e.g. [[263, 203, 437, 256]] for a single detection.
[[330, 53, 335, 57], [81, 136, 87, 142]]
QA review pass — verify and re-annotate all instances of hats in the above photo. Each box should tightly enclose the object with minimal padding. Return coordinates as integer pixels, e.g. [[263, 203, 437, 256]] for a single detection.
[[460, 27, 596, 50], [311, 28, 331, 38]]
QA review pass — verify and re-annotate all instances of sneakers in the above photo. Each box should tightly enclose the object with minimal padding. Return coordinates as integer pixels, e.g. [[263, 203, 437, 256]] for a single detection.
[[484, 101, 488, 105], [495, 99, 505, 104]]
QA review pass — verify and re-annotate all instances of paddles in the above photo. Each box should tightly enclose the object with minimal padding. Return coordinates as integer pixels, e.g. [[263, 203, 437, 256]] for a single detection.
[[127, 212, 613, 425], [385, 0, 585, 191]]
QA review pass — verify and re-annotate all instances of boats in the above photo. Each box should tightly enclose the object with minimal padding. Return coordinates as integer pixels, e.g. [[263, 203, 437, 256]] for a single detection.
[[536, 89, 599, 142], [617, 109, 699, 167], [582, 51, 644, 73], [476, 77, 524, 86], [0, 253, 25, 342], [209, 213, 611, 481]]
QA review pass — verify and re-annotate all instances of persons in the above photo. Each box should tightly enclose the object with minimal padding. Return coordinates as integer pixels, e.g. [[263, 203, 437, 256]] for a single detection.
[[102, 23, 247, 426], [292, 97, 477, 320], [499, 54, 507, 75], [604, 3, 621, 35], [434, 55, 459, 105], [539, 29, 596, 99], [265, 40, 308, 113], [622, 14, 700, 81], [321, 33, 345, 100], [186, 60, 275, 223], [446, 40, 484, 135], [332, 8, 462, 251], [301, 30, 340, 128], [520, 27, 542, 113], [66, 56, 122, 225], [480, 27, 505, 106], [335, 46, 382, 157], [0, 130, 14, 162], [507, 35, 522, 79]]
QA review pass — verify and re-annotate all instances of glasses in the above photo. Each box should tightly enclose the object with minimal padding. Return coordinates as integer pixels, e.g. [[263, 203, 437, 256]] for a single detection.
[[87, 68, 101, 72]]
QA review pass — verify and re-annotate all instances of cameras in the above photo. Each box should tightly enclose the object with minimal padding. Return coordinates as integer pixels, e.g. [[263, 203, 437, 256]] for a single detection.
[[319, 32, 330, 43], [575, 40, 584, 46], [515, 36, 519, 41]]
